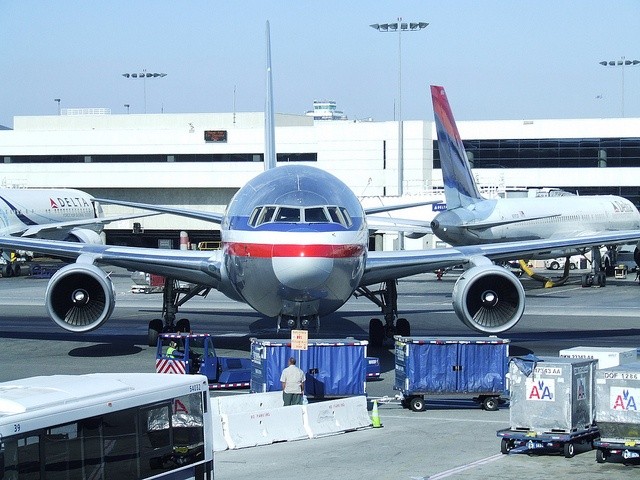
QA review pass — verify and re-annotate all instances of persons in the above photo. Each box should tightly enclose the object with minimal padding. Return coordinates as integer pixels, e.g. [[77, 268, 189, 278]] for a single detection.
[[280, 357, 307, 407], [166, 341, 185, 358]]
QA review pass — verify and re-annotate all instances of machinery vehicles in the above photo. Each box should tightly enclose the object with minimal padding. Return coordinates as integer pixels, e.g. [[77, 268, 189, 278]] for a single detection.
[[156, 332, 381, 398]]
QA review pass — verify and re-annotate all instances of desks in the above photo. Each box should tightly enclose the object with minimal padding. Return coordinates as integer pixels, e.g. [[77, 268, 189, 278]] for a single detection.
[[614, 268, 627, 279]]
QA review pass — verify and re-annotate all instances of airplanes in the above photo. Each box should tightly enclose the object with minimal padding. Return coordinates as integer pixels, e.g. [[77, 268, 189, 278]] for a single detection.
[[0, 21, 638, 348], [364, 84, 639, 289], [0, 184, 167, 278]]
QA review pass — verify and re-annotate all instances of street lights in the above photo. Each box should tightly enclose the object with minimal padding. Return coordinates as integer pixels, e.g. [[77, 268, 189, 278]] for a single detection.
[[369, 19, 430, 195], [600, 56, 639, 119], [121, 69, 168, 114]]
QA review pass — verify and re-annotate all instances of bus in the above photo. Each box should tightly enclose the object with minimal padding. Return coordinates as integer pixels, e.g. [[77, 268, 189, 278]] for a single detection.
[[0, 372, 214, 480]]
